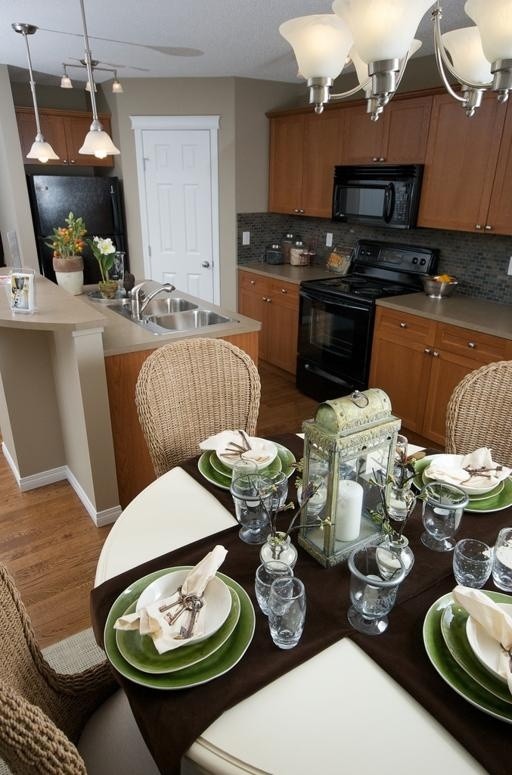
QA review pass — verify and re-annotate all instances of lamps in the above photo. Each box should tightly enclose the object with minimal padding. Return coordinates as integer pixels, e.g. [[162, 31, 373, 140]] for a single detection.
[[59, 61, 124, 95], [275, 0, 511, 119], [11, 21, 62, 164], [76, 0, 124, 161]]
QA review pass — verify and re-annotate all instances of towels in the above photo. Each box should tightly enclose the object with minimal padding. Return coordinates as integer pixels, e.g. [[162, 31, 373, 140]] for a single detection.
[[197, 425, 272, 466], [420, 447, 506, 491], [112, 542, 231, 657], [452, 581, 512, 700]]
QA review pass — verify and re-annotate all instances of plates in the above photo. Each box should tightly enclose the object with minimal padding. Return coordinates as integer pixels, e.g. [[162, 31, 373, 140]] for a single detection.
[[196, 437, 298, 490], [103, 565, 257, 690], [422, 588, 511, 725], [410, 453, 512, 517]]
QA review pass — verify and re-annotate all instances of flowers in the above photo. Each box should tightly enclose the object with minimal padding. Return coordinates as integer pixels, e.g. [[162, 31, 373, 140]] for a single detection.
[[43, 208, 87, 258], [248, 445, 433, 585], [87, 233, 120, 284]]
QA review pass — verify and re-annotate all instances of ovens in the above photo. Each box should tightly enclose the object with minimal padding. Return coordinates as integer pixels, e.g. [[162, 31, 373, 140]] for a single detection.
[[294, 293, 376, 404]]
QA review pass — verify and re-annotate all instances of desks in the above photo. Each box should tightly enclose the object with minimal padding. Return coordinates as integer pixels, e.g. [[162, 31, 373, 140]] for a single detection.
[[1, 263, 122, 528]]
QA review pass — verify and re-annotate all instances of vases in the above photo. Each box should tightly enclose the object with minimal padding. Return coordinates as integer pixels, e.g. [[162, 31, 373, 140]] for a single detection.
[[98, 280, 118, 301], [51, 255, 86, 296]]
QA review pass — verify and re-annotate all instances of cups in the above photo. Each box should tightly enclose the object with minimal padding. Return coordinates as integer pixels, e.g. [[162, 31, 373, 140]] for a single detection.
[[104, 250, 125, 293], [452, 527, 512, 594], [394, 435, 409, 474], [255, 561, 307, 654]]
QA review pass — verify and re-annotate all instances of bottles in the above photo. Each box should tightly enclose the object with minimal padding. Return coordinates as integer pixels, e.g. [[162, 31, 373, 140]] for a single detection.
[[266, 233, 311, 266]]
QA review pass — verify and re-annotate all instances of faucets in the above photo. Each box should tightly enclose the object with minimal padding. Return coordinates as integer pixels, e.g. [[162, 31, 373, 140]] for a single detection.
[[126, 280, 176, 318]]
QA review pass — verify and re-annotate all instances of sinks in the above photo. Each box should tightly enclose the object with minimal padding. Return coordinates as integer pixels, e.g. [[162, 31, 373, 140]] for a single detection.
[[143, 298, 199, 317], [148, 309, 232, 332]]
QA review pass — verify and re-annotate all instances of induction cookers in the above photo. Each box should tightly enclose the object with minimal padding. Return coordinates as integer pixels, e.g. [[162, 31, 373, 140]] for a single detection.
[[301, 274, 422, 301]]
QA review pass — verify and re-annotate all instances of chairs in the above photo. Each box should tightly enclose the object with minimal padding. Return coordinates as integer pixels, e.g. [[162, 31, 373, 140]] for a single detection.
[[1, 558, 160, 775], [443, 358, 511, 472], [133, 337, 262, 483]]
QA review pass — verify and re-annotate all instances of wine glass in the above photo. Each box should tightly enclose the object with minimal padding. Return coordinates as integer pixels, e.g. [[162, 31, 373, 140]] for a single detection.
[[344, 543, 407, 638], [420, 483, 469, 551], [232, 460, 287, 545]]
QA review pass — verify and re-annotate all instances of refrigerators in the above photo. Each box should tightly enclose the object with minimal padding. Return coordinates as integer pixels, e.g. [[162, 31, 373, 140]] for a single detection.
[[31, 173, 130, 286]]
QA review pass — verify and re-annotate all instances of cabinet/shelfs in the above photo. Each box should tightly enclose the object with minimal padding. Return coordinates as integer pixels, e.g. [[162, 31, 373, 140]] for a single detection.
[[343, 89, 434, 166], [15, 105, 115, 168], [416, 87, 511, 236], [263, 108, 343, 218], [368, 298, 512, 445], [237, 265, 300, 377]]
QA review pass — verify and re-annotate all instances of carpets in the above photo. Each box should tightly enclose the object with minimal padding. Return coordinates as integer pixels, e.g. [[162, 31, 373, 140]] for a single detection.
[[0, 627, 109, 775]]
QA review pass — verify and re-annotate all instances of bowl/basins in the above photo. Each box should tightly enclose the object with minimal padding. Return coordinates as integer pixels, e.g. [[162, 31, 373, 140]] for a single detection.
[[423, 275, 460, 298]]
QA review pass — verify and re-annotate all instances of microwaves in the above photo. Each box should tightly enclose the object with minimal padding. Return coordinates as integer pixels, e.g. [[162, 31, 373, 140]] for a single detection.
[[330, 164, 424, 232]]
